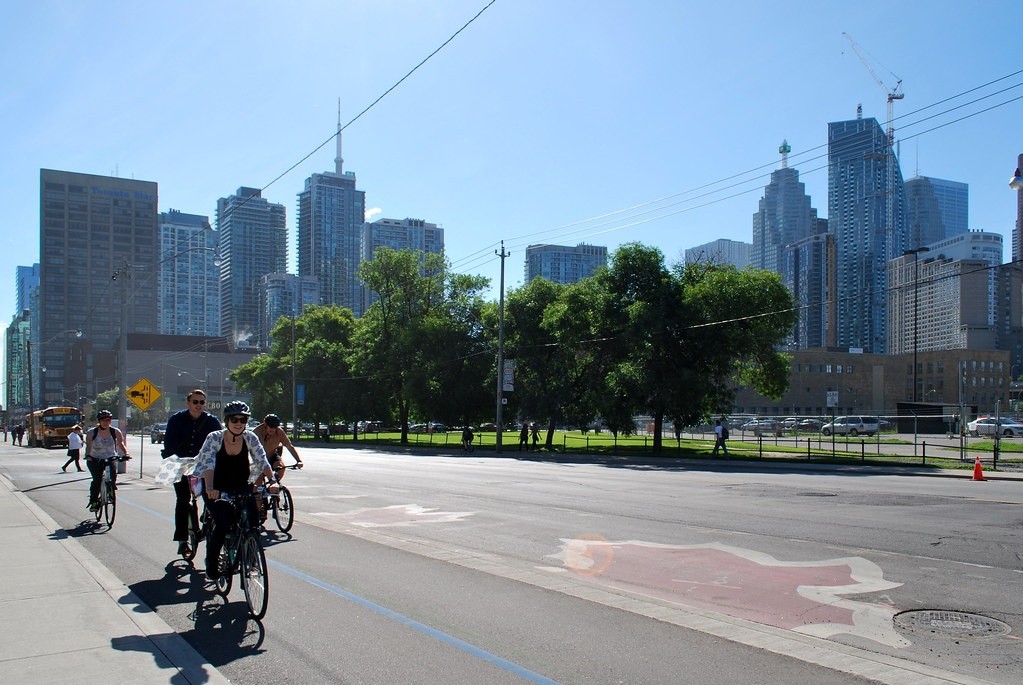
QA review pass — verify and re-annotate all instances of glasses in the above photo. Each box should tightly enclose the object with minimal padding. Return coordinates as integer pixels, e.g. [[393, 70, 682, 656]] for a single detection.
[[227, 416, 249, 425], [268, 424, 278, 429], [187, 399, 208, 405]]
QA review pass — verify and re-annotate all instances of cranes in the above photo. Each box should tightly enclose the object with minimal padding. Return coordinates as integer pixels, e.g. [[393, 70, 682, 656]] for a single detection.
[[842, 30, 906, 261]]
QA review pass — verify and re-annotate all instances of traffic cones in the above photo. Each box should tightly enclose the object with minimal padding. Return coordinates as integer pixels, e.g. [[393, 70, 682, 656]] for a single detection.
[[969, 455, 987, 482]]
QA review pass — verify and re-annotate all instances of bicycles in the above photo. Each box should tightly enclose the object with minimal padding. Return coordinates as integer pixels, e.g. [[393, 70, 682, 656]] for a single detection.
[[205, 485, 285, 622], [82, 455, 132, 526], [162, 457, 303, 562]]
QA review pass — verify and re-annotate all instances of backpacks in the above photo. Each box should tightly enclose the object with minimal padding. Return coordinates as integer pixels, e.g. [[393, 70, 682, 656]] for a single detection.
[[721, 426, 729, 439]]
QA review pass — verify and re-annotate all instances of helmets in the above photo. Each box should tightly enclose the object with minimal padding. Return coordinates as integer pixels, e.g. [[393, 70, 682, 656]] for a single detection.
[[264, 414, 281, 429], [222, 400, 252, 417], [96, 409, 113, 421]]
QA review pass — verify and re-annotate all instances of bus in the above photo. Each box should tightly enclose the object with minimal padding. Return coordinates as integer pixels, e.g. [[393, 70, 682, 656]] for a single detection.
[[25, 406, 86, 449]]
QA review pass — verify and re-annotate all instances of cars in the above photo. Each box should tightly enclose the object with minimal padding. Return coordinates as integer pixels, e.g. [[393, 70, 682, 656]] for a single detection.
[[821, 415, 881, 438], [965, 416, 1023, 438], [719, 414, 828, 432], [244, 420, 502, 435], [150, 423, 167, 444]]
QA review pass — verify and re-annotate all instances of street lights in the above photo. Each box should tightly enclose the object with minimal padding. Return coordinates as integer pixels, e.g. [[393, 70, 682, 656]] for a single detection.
[[901, 246, 932, 404], [26, 328, 85, 448], [177, 371, 209, 411], [111, 243, 223, 475]]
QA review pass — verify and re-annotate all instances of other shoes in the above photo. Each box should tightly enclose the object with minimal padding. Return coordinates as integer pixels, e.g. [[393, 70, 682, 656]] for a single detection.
[[177, 543, 192, 554], [89, 501, 99, 512], [204, 557, 218, 580], [78, 469, 85, 472], [62, 467, 66, 472]]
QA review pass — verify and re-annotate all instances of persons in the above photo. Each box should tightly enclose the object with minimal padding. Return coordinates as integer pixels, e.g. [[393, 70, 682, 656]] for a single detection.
[[528, 424, 542, 453], [85, 410, 132, 512], [711, 420, 730, 458], [160, 389, 225, 554], [62, 426, 85, 472], [462, 424, 474, 451], [12, 426, 18, 445], [250, 413, 303, 512], [156, 401, 280, 578], [17, 424, 24, 446], [518, 423, 530, 452]]
